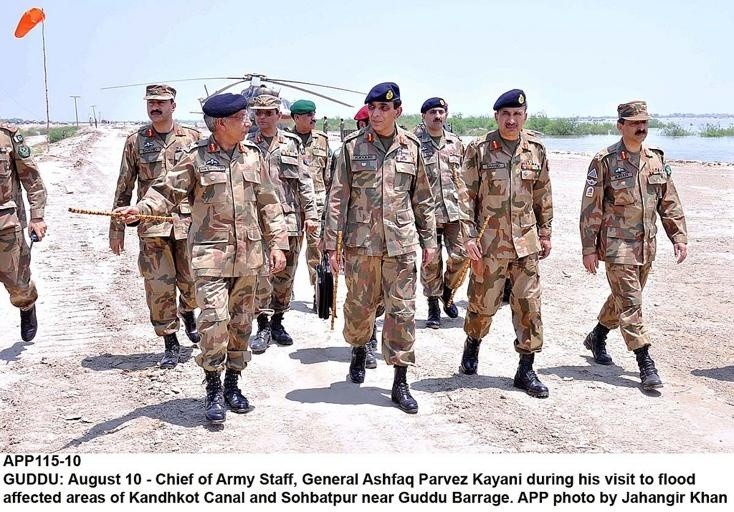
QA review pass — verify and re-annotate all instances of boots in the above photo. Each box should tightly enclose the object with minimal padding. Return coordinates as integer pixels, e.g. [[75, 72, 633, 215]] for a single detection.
[[425, 284, 458, 328], [178, 308, 202, 343], [390, 365, 420, 415], [159, 332, 181, 368], [632, 345, 664, 391], [224, 366, 249, 414], [349, 323, 380, 384], [269, 312, 294, 347], [583, 322, 615, 365], [202, 369, 225, 424], [250, 310, 272, 353], [461, 333, 481, 374], [513, 352, 550, 398]]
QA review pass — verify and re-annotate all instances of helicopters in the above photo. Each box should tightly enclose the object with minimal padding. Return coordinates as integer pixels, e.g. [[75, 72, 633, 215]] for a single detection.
[[101, 73, 370, 135]]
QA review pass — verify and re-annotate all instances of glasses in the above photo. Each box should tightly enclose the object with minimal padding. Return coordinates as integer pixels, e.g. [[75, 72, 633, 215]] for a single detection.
[[254, 110, 273, 116]]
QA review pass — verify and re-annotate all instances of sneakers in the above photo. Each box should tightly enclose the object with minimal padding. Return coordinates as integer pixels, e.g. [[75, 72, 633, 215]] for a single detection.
[[17, 300, 40, 342]]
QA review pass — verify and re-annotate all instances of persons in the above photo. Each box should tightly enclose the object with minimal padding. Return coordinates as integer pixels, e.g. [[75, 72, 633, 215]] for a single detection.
[[0, 122, 48, 342], [579, 101, 688, 391]]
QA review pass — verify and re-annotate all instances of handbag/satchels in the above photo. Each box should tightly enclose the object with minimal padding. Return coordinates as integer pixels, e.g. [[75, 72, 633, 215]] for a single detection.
[[315, 263, 335, 320]]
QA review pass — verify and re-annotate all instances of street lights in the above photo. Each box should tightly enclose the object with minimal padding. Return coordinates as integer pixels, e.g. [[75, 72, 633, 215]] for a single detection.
[[70, 95, 81, 128], [90, 105, 97, 119]]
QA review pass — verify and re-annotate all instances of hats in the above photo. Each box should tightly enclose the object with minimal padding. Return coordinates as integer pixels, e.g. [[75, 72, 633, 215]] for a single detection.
[[420, 96, 448, 115], [144, 84, 177, 102], [251, 95, 281, 112], [618, 102, 649, 123], [203, 92, 246, 120], [491, 88, 526, 109], [289, 99, 317, 114], [354, 81, 402, 121]]
